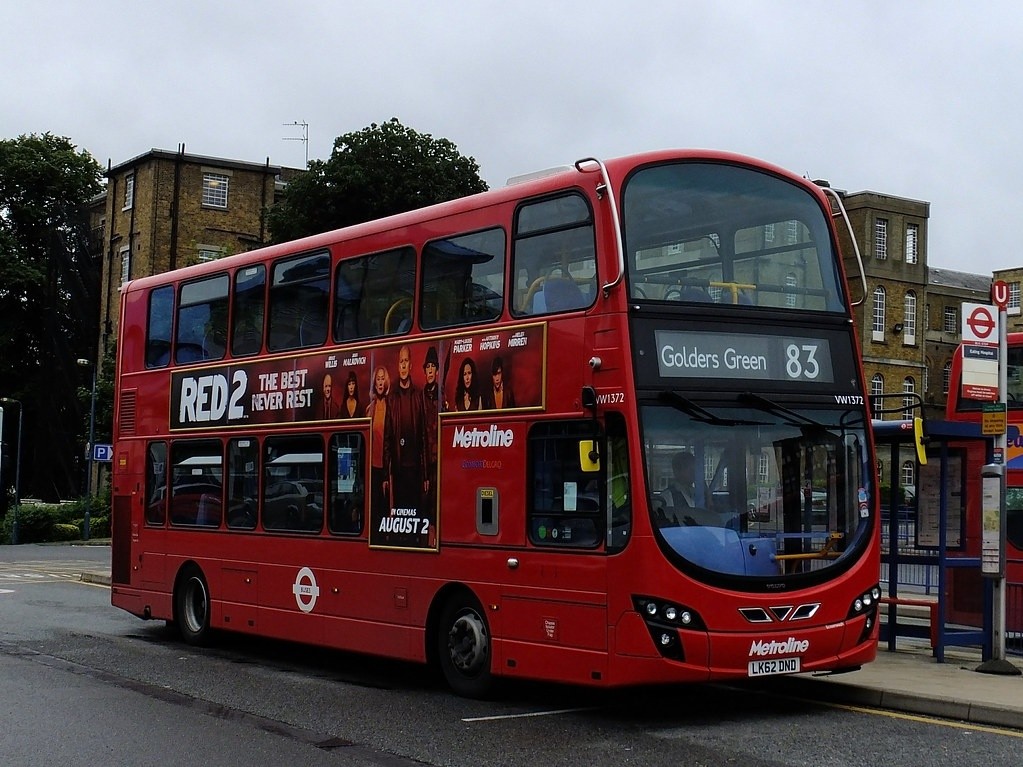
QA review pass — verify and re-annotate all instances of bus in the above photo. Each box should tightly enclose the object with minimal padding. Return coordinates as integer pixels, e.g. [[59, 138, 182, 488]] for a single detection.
[[944, 333, 1023, 634]]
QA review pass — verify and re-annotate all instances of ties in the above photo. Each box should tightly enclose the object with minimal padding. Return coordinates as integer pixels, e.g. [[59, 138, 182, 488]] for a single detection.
[[325, 397, 329, 419]]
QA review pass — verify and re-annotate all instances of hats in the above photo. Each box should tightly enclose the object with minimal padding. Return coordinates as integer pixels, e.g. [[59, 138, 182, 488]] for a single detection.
[[423, 346, 439, 370], [672, 452, 696, 467]]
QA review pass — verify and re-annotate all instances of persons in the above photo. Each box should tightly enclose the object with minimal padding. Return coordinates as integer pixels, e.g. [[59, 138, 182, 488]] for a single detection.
[[320, 346, 514, 547], [651, 452, 709, 527]]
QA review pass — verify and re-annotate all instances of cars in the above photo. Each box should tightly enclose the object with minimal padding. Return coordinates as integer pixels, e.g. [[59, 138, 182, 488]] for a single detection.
[[878, 482, 916, 508], [746, 489, 828, 524], [110, 149, 930, 702], [243, 478, 326, 509], [146, 481, 222, 527]]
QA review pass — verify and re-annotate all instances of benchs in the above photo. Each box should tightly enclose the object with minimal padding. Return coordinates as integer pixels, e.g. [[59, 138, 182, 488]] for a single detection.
[[879, 591, 939, 657]]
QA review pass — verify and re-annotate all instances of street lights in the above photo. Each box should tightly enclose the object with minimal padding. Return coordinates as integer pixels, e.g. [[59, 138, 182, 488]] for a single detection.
[[0, 398, 23, 545], [78, 359, 97, 542]]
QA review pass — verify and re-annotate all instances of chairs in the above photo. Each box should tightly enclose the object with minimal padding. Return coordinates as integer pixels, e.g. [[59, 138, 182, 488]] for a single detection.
[[681, 276, 714, 302], [336, 299, 361, 338], [233, 320, 261, 354], [300, 311, 327, 346], [543, 266, 585, 311], [201, 328, 225, 360], [148, 340, 170, 364], [384, 298, 413, 334], [720, 280, 752, 305]]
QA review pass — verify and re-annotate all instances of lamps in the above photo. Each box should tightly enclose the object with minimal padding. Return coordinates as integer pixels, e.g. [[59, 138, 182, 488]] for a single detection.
[[893, 323, 904, 335]]
